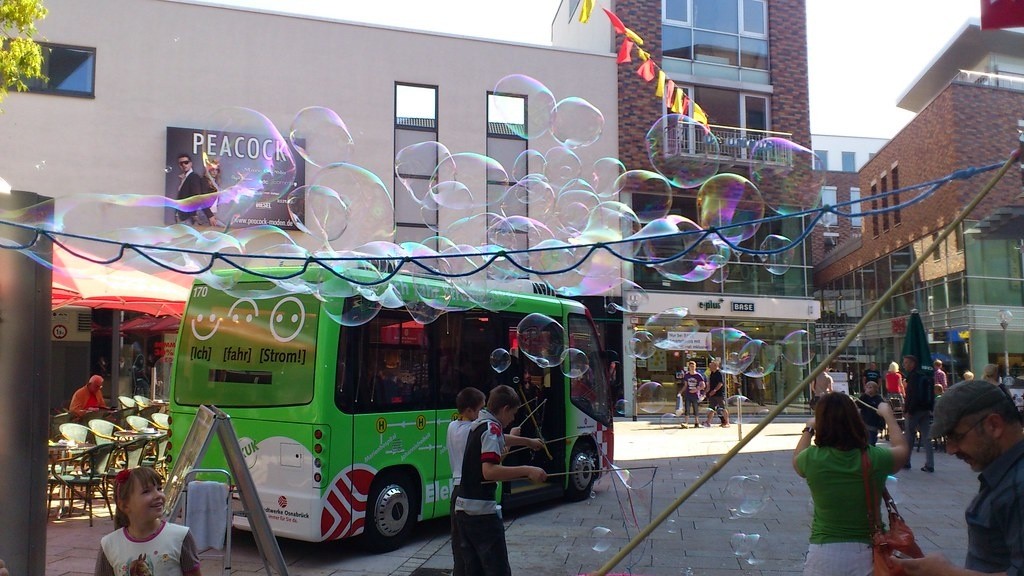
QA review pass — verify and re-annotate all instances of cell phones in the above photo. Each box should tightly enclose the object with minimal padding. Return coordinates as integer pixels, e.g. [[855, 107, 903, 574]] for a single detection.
[[892, 549, 915, 559]]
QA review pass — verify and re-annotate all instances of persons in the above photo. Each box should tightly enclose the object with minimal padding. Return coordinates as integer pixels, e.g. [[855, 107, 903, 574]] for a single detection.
[[752, 367, 766, 406], [889, 380, 1024, 576], [792, 392, 909, 576], [175, 154, 220, 226], [676, 361, 706, 428], [69, 375, 105, 416], [811, 368, 833, 396], [95, 467, 202, 576], [853, 356, 1012, 472], [701, 360, 730, 427], [446, 385, 549, 576]]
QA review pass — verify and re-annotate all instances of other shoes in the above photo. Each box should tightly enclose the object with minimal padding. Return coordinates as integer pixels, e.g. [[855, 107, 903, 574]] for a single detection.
[[920, 466, 934, 473], [902, 463, 912, 468], [700, 421, 710, 427], [681, 423, 689, 429], [720, 423, 730, 428], [694, 423, 700, 427]]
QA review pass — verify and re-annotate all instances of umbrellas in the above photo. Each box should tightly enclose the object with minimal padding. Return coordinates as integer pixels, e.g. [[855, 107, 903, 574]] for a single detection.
[[899, 309, 934, 377], [110, 314, 180, 374], [52, 239, 190, 315]]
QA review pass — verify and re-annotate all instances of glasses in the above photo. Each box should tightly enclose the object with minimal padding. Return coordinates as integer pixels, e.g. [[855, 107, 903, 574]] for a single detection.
[[945, 415, 987, 443]]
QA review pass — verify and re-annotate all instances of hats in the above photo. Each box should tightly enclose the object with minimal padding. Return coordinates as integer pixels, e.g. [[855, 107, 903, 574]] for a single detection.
[[929, 381, 1006, 438]]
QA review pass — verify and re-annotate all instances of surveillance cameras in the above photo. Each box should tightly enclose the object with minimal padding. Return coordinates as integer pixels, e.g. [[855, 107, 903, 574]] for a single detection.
[[629, 302, 639, 311], [837, 331, 846, 336]]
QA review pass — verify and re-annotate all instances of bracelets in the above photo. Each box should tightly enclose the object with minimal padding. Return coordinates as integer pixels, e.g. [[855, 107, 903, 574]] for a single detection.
[[714, 389, 716, 392]]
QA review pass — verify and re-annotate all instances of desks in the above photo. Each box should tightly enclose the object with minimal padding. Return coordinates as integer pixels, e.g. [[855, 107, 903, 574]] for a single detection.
[[48, 443, 95, 526]]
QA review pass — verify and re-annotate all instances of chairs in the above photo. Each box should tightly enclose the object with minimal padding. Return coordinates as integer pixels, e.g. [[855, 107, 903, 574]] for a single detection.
[[48, 395, 172, 527]]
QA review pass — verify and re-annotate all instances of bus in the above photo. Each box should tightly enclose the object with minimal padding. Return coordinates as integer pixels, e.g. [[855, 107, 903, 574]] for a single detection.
[[166, 267, 614, 552]]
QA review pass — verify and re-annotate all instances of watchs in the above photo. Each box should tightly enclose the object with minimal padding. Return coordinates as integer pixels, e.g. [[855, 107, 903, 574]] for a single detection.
[[802, 427, 814, 435]]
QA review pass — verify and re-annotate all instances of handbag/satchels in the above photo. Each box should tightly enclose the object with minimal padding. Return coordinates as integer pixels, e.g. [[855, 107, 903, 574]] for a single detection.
[[861, 449, 926, 576]]
